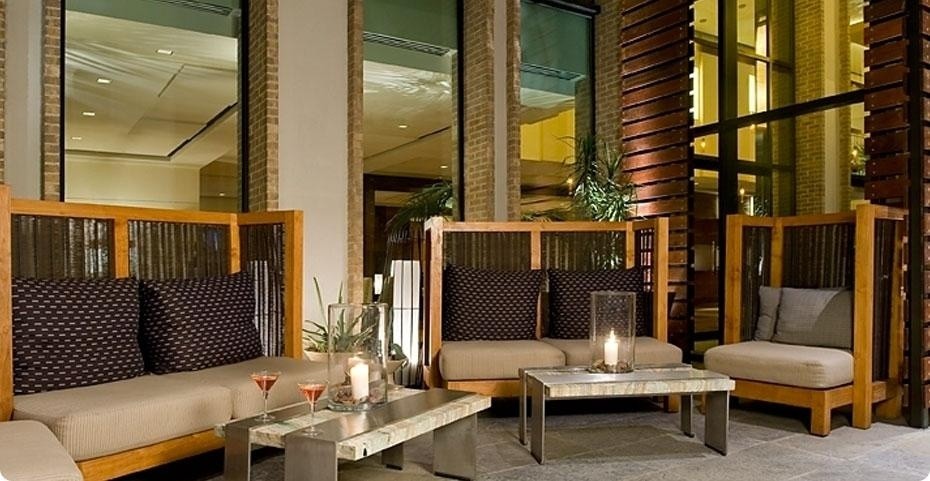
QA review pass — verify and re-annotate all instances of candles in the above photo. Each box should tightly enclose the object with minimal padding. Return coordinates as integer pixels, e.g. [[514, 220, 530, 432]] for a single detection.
[[350, 361, 370, 399], [604, 327, 619, 365], [348, 355, 361, 373]]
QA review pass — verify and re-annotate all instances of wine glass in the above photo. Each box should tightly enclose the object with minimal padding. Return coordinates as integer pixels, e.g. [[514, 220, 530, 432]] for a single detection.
[[249, 372, 279, 421], [296, 380, 328, 436]]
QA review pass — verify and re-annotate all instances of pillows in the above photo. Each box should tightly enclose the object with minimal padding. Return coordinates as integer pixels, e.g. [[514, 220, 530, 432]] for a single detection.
[[11, 275, 145, 395], [141, 270, 263, 374], [754, 285, 783, 340], [443, 262, 544, 339], [548, 265, 649, 338], [772, 287, 852, 347]]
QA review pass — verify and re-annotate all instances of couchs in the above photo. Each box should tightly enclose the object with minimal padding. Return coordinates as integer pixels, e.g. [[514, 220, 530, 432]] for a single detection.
[[1, 419, 84, 481], [422, 216, 683, 412], [700, 203, 907, 437], [0, 181, 345, 481]]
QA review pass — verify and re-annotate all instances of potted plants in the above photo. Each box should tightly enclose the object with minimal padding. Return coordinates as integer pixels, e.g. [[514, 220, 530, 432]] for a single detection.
[[301, 276, 380, 362], [387, 336, 408, 385]]
